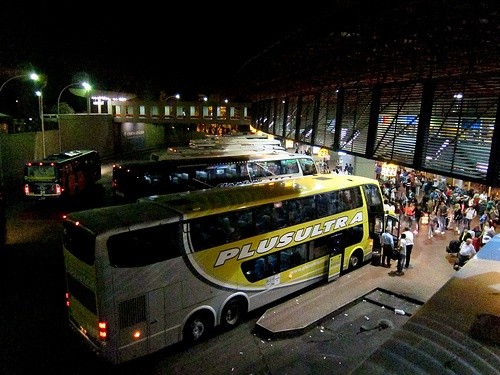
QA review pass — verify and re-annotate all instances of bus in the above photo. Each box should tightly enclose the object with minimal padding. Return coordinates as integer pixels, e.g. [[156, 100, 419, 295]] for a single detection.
[[112, 154, 318, 206], [60, 174, 400, 365], [22, 150, 102, 201]]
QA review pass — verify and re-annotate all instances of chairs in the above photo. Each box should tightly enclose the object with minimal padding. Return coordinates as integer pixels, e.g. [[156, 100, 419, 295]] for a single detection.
[[253, 248, 294, 280], [214, 211, 315, 240]]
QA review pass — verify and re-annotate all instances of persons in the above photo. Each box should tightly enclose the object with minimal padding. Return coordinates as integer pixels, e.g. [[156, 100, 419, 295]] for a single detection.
[[114, 140, 500, 282]]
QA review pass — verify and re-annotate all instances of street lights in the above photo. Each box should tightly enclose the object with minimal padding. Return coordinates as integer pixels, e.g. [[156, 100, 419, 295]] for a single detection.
[[57, 81, 91, 151], [35, 90, 46, 160], [0, 72, 41, 93]]
